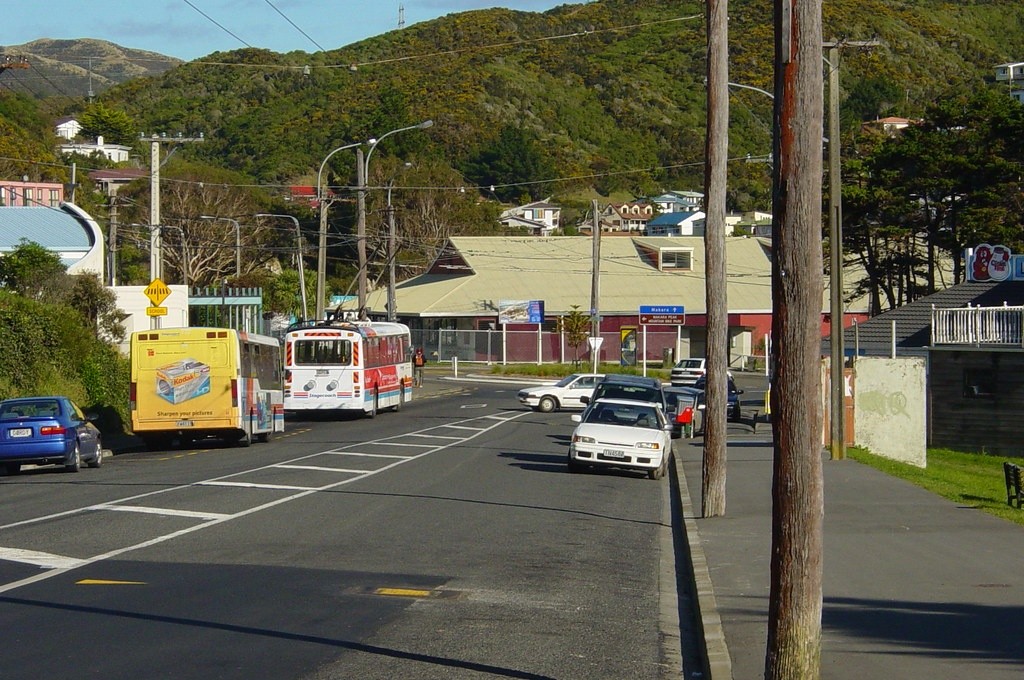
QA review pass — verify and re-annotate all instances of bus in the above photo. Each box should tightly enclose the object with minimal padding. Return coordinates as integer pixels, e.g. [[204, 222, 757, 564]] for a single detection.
[[284, 321, 412, 419], [130, 327, 284, 450]]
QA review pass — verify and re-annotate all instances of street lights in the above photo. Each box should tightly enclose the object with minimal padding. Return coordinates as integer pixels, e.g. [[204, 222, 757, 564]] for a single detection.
[[357, 120, 433, 310], [201, 215, 240, 278], [386, 162, 412, 322], [316, 138, 376, 326], [256, 214, 309, 327], [133, 223, 188, 284]]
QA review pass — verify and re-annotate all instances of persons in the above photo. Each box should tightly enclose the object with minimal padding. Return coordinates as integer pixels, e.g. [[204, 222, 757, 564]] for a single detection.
[[412, 348, 426, 388]]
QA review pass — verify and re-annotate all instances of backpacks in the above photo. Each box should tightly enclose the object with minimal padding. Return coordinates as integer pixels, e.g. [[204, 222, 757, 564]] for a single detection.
[[416, 354, 422, 365]]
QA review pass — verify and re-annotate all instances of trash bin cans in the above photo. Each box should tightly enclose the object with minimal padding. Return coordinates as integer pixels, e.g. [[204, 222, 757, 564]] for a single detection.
[[676, 395, 695, 424]]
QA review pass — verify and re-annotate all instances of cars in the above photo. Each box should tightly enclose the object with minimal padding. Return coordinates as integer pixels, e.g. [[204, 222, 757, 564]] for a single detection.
[[694, 375, 744, 422], [0, 396, 102, 476], [580, 374, 675, 422], [671, 358, 735, 387], [515, 374, 606, 413], [568, 397, 673, 480]]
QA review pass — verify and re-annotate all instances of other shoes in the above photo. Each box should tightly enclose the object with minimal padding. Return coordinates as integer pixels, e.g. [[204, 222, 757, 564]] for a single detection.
[[415, 381, 417, 385], [420, 386, 423, 388]]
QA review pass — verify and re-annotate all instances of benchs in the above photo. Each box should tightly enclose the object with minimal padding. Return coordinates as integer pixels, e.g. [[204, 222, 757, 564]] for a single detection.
[[1004, 461, 1024, 509]]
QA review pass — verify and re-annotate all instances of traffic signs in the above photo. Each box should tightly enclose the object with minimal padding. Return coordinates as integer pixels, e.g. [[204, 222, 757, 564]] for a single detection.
[[639, 305, 685, 325]]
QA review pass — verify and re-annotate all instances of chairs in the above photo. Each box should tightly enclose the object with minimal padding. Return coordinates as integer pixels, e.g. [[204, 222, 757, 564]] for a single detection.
[[1, 413, 19, 418], [38, 411, 53, 417], [600, 409, 614, 421], [605, 389, 621, 398], [631, 413, 653, 427]]
